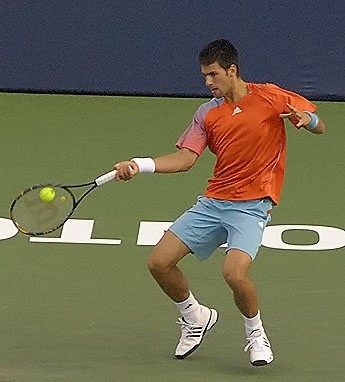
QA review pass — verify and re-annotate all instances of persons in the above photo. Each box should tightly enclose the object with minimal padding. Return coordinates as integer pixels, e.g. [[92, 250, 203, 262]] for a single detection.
[[113, 40, 325, 365]]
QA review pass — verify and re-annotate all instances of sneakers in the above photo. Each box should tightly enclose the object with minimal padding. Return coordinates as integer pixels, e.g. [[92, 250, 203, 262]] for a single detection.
[[244, 325, 274, 366], [174, 305, 218, 359]]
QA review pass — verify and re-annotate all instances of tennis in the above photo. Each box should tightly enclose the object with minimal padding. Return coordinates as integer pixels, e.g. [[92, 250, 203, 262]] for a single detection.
[[39, 187, 55, 202]]
